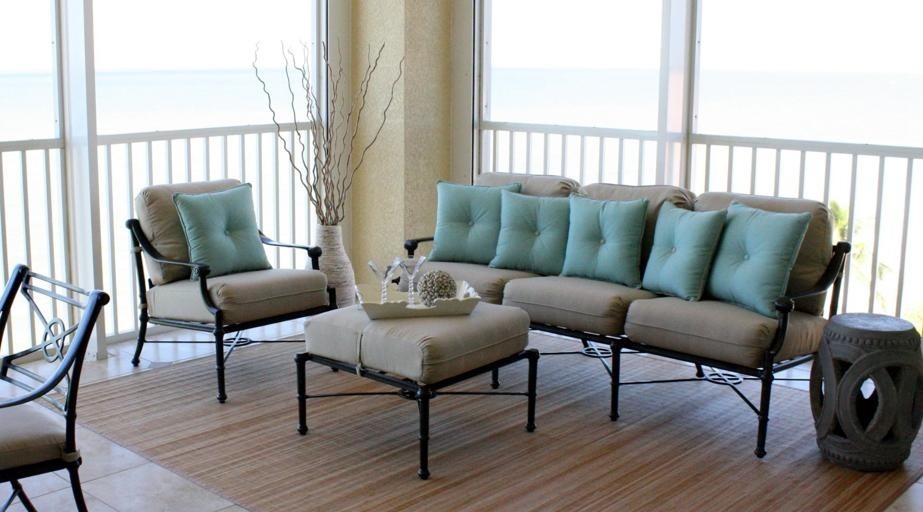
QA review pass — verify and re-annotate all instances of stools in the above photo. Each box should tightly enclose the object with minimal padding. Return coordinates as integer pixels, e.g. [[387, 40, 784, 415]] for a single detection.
[[809, 312, 922, 472]]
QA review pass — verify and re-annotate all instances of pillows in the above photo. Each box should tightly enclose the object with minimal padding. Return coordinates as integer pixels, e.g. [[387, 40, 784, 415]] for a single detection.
[[707, 196, 814, 317], [641, 199, 728, 302], [171, 183, 273, 282], [427, 180, 522, 265], [696, 191, 833, 317], [488, 188, 571, 276], [560, 193, 650, 289]]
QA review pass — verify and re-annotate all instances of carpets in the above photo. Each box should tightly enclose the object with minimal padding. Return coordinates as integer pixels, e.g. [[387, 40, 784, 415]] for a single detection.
[[32, 332, 923, 512]]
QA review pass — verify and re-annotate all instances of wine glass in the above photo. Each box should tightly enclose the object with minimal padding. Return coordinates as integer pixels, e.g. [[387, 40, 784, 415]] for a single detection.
[[368, 257, 399, 304], [397, 254, 426, 306]]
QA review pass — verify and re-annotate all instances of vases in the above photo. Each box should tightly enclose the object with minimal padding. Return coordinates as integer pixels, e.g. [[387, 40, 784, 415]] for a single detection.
[[302, 223, 356, 309]]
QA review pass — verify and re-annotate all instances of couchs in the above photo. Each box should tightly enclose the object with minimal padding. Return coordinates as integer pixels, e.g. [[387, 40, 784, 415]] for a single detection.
[[395, 171, 851, 458]]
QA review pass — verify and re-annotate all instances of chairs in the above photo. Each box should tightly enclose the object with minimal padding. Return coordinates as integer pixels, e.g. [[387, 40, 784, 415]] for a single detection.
[[126, 178, 340, 404], [0, 263, 110, 512]]
